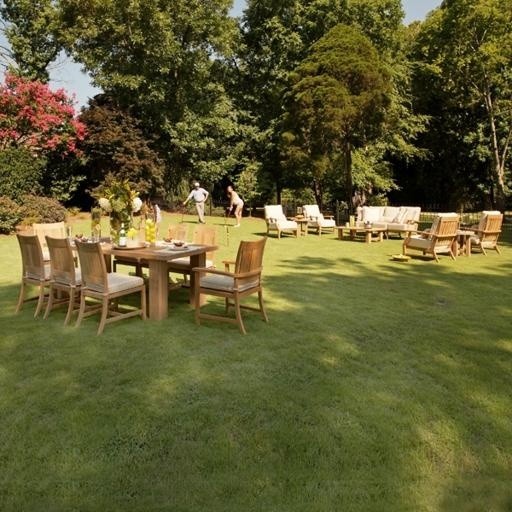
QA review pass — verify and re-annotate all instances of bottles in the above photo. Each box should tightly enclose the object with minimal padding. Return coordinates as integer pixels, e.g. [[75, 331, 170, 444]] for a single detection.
[[118, 221, 127, 246]]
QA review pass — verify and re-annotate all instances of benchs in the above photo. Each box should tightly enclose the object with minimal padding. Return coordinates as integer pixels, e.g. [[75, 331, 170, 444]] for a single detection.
[[356, 206, 421, 239]]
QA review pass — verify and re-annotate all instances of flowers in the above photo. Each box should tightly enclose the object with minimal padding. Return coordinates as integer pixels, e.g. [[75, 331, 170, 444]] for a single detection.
[[86, 170, 143, 241]]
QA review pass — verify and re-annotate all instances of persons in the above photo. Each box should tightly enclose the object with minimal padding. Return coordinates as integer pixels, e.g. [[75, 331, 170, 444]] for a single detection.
[[225, 185, 245, 227], [182, 183, 209, 223]]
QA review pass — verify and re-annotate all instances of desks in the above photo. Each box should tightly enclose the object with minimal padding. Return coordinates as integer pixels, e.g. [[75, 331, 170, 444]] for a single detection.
[[423, 228, 474, 257]]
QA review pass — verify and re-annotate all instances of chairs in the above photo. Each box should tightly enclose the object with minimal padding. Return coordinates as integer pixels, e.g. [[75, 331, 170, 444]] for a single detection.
[[403, 215, 457, 264], [459, 210, 504, 257], [264, 204, 298, 240], [16, 220, 267, 337], [302, 204, 336, 237]]
